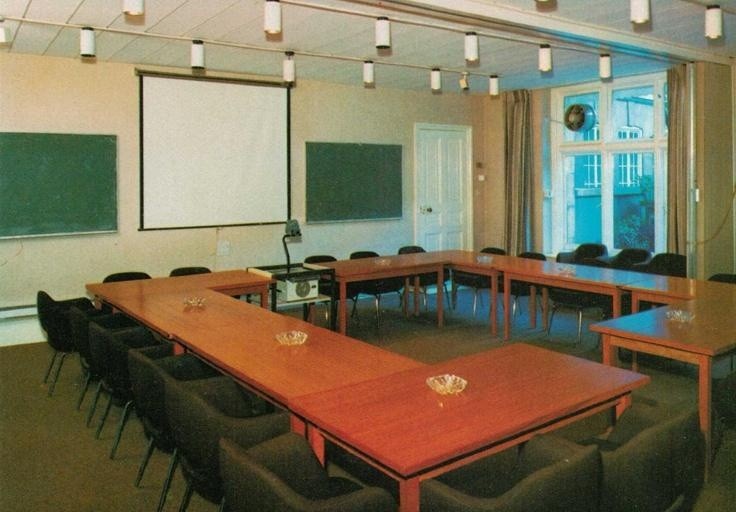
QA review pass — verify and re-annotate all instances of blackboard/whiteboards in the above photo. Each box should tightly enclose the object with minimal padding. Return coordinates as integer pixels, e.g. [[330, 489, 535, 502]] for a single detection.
[[0, 129, 122, 241], [303, 140, 406, 226]]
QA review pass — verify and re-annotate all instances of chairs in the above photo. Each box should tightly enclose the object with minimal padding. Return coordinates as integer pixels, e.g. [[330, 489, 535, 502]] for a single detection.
[[34, 248, 736, 512]]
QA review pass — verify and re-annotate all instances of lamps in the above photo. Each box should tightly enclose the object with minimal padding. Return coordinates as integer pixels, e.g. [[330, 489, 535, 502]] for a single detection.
[[362, 59, 375, 86], [78, 26, 99, 59], [262, 2, 283, 36], [536, 42, 554, 73], [627, 1, 651, 26], [189, 38, 207, 72], [597, 52, 613, 80], [373, 15, 393, 51], [462, 30, 480, 64], [428, 68, 443, 93], [120, 1, 145, 17], [486, 74, 500, 96], [703, 3, 722, 42], [282, 51, 297, 84]]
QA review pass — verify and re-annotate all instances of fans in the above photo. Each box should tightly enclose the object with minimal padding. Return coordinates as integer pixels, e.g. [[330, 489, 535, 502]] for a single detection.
[[544, 103, 596, 132]]
